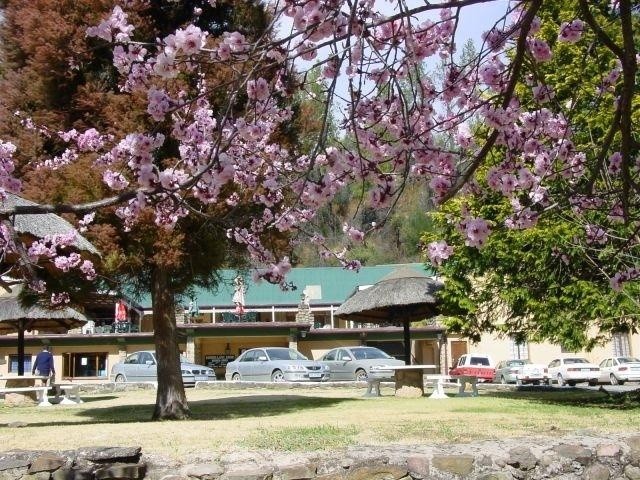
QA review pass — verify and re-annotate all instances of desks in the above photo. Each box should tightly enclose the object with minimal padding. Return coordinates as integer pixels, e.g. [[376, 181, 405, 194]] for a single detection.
[[379, 364, 437, 397], [0, 375, 47, 405]]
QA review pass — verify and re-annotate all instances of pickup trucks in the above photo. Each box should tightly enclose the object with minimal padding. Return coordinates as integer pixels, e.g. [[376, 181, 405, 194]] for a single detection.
[[448, 354, 496, 383]]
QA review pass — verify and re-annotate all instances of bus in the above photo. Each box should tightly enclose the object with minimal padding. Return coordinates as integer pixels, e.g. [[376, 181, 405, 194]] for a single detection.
[[600, 356, 640, 386]]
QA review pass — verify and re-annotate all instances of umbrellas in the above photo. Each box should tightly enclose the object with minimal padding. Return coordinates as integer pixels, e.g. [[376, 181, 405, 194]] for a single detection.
[[232, 276, 245, 322]]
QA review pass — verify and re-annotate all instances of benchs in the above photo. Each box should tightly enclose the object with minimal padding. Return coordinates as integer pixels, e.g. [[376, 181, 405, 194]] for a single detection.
[[424, 374, 480, 399], [363, 377, 396, 398], [0, 386, 53, 406], [53, 383, 83, 405]]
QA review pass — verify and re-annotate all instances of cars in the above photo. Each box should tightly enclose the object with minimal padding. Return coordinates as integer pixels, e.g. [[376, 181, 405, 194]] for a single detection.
[[228, 346, 331, 383], [545, 357, 600, 387], [112, 350, 217, 389], [316, 345, 406, 384], [494, 360, 550, 387]]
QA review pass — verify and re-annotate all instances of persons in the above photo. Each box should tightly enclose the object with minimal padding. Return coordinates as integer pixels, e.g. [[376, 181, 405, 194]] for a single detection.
[[31, 344, 56, 404]]
